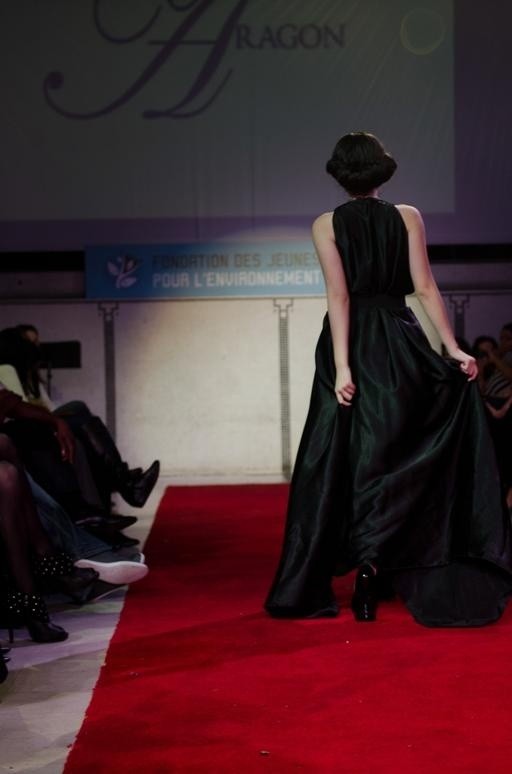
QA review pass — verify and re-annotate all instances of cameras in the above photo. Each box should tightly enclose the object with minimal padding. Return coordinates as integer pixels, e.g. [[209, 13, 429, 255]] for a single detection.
[[476, 351, 487, 358]]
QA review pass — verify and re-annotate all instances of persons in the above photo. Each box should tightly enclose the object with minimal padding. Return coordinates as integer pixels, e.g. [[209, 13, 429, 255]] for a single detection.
[[306, 131, 486, 624], [435, 325, 511, 443]]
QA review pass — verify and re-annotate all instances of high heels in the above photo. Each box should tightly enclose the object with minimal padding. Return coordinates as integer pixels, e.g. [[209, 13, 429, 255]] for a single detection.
[[9, 460, 159, 642], [351, 567, 379, 625]]
